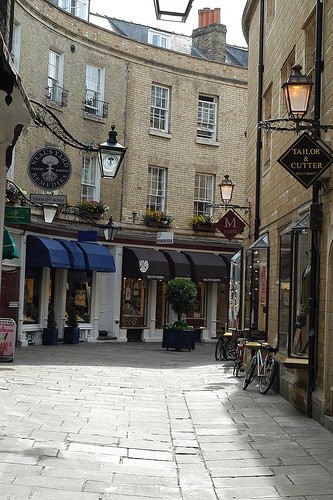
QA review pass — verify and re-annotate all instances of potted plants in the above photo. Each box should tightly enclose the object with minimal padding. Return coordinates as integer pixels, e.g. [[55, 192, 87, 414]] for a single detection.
[[42, 303, 59, 346], [63, 309, 80, 344], [162, 277, 198, 352]]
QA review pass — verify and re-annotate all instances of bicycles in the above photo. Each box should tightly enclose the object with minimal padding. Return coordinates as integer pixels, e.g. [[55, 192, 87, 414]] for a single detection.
[[243, 341, 277, 393], [215, 327, 246, 377]]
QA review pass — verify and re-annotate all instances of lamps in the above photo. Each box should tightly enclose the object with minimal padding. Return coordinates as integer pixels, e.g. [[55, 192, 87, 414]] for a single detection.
[[257, 63, 314, 135], [60, 205, 120, 242], [30, 99, 128, 179], [206, 175, 252, 240], [6, 179, 59, 225]]
